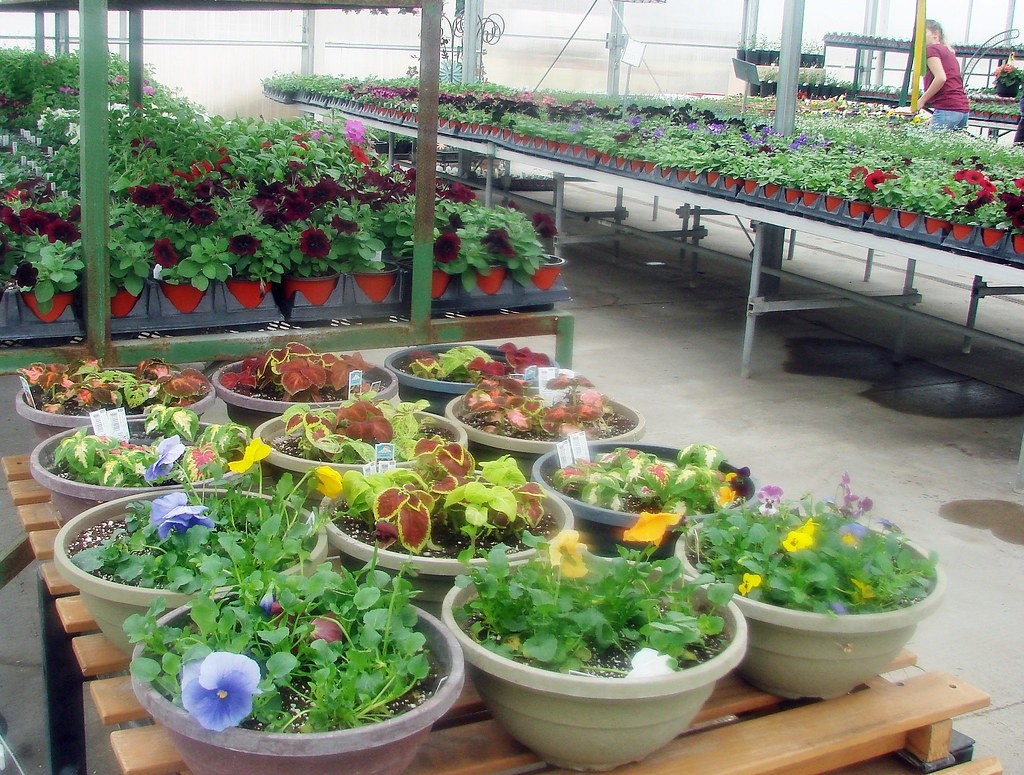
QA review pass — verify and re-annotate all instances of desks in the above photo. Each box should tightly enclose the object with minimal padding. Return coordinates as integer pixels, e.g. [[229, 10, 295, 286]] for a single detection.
[[0, 452, 1010, 775]]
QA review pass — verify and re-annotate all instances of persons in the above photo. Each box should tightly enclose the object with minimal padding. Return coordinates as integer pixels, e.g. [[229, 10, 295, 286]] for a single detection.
[[914, 19, 970, 133], [1013, 90, 1024, 143]]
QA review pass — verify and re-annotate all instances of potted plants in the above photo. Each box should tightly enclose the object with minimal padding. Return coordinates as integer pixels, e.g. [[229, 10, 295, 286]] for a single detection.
[[733, 32, 1024, 121]]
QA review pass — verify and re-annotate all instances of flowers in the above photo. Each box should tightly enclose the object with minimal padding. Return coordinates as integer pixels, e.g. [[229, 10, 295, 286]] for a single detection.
[[117, 496, 434, 736], [992, 64, 1023, 90], [73, 434, 343, 592], [2, 174, 571, 269], [450, 512, 736, 681], [669, 471, 939, 624]]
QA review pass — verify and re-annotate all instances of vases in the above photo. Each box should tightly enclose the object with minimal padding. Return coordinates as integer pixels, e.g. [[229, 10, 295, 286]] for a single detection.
[[364, 103, 1024, 254], [22, 256, 945, 775], [997, 84, 1018, 98]]
